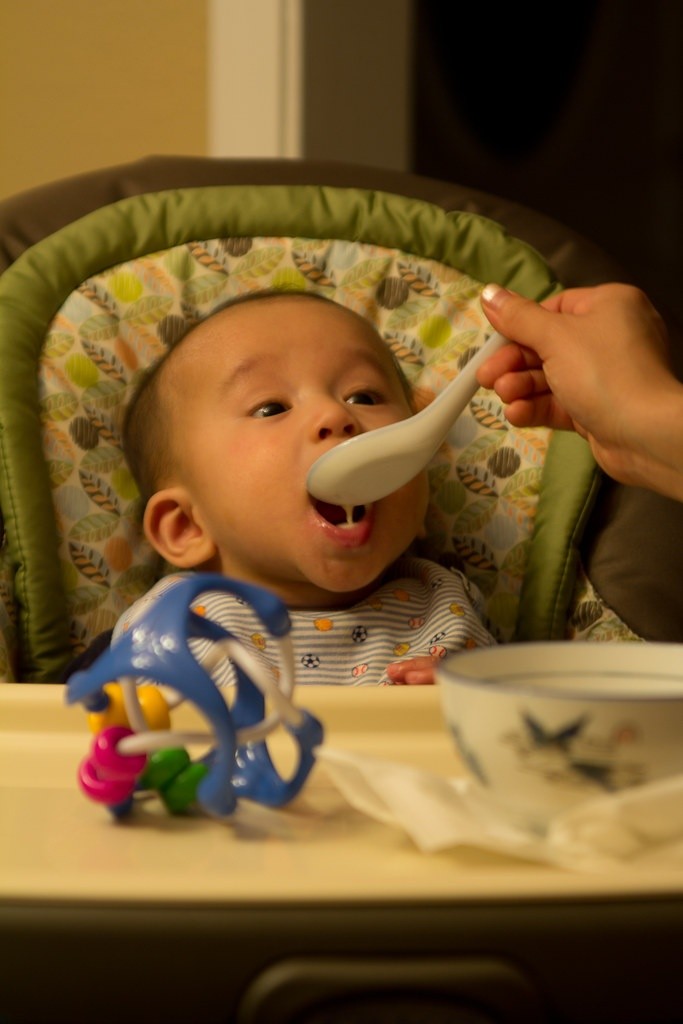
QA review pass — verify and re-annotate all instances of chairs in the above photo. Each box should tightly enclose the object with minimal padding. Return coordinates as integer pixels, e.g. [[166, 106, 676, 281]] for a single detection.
[[0, 150, 683, 684]]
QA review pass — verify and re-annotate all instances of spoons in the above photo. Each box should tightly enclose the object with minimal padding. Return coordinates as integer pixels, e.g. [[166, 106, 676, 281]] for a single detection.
[[305, 332, 509, 506]]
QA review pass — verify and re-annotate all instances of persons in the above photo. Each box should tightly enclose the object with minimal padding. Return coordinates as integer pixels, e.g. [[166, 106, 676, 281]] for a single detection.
[[476, 277, 683, 501], [106, 282, 499, 687]]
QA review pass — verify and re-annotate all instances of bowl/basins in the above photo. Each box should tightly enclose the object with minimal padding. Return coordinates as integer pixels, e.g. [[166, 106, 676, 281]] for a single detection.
[[435, 640, 683, 838]]
